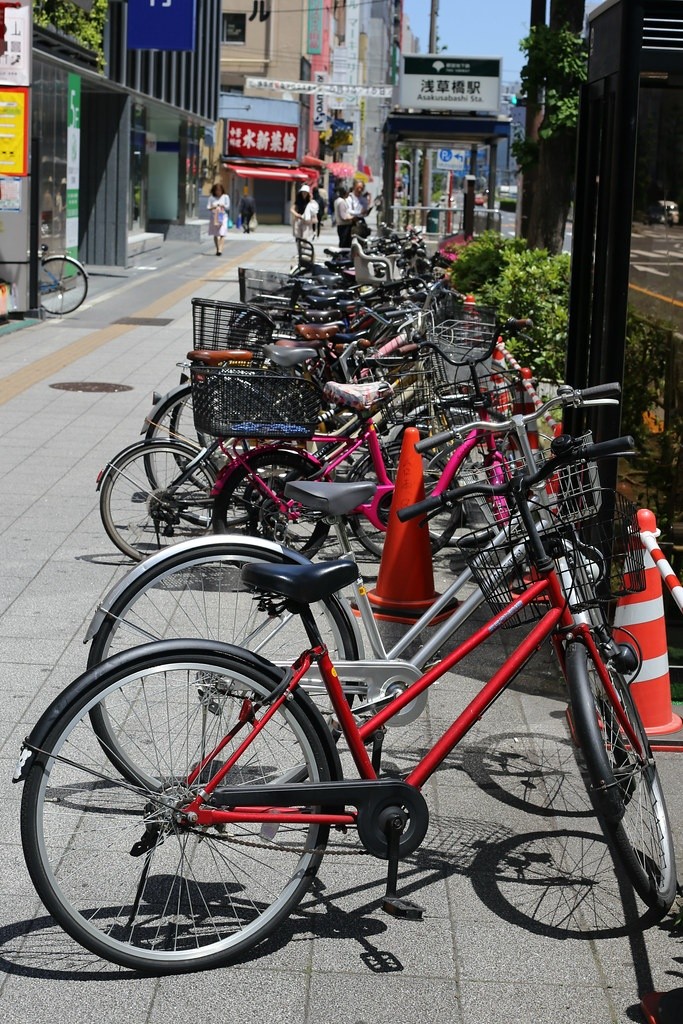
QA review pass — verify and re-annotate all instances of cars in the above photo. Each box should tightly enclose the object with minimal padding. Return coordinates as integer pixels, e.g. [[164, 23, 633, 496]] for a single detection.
[[475, 194, 484, 205]]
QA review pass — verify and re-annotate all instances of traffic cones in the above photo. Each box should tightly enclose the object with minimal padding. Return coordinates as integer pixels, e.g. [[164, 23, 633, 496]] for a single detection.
[[566, 509, 683, 751], [351, 428, 464, 625]]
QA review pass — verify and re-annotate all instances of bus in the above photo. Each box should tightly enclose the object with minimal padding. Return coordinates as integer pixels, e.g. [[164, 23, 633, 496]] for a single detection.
[[652, 200, 679, 224]]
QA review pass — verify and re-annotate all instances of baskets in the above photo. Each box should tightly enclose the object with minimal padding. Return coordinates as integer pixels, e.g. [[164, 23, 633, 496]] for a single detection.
[[187, 267, 647, 631]]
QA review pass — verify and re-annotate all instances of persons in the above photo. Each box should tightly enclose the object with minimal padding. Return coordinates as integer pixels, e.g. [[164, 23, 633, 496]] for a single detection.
[[329, 179, 371, 248], [239, 186, 256, 235], [394, 186, 404, 205], [291, 185, 324, 261], [207, 183, 230, 256], [44, 176, 67, 251], [374, 189, 385, 229], [439, 193, 447, 208]]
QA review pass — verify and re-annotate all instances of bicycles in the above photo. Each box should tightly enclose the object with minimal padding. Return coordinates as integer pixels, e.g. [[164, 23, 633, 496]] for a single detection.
[[97, 222, 536, 568], [28, 243, 90, 319], [82, 380, 623, 794], [13, 434, 678, 971]]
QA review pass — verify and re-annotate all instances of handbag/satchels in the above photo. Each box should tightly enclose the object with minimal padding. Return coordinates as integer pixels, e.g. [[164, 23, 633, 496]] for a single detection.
[[212, 206, 223, 225], [228, 219, 233, 228], [249, 214, 258, 229], [351, 218, 371, 237], [236, 214, 243, 228]]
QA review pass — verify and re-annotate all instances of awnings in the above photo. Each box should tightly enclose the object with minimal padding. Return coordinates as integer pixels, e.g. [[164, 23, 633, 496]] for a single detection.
[[223, 163, 309, 181]]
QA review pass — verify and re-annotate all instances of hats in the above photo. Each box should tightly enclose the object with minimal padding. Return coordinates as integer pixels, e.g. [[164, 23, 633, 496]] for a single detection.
[[298, 185, 310, 193]]
